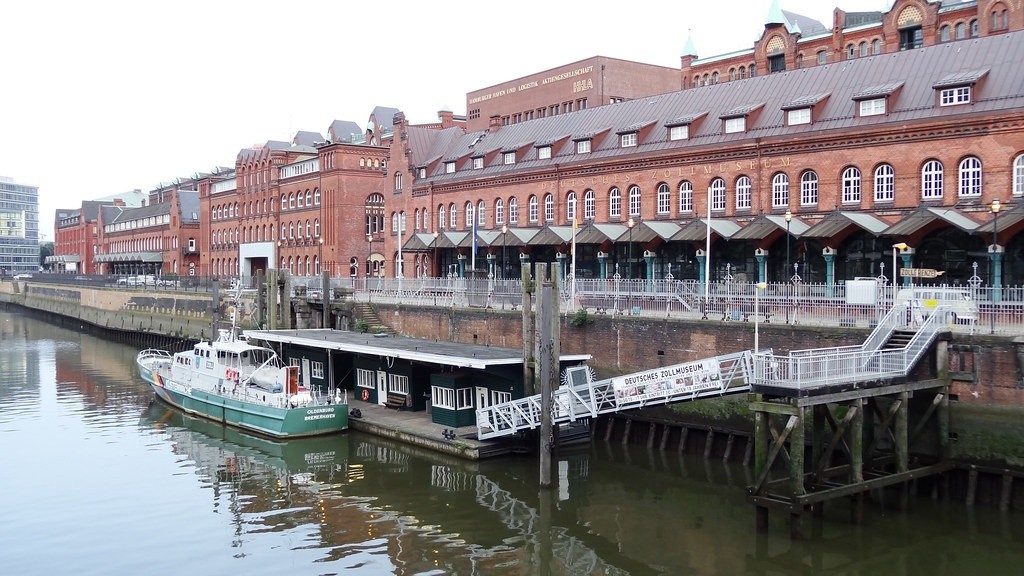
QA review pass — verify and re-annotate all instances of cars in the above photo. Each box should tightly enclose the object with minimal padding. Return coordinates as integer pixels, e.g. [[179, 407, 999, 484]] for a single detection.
[[118, 276, 175, 286], [74, 275, 87, 281], [14, 273, 32, 281]]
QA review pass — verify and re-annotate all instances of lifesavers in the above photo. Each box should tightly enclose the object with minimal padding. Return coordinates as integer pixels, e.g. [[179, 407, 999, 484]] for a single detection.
[[361, 389, 370, 401], [228, 370, 237, 381], [225, 369, 231, 380]]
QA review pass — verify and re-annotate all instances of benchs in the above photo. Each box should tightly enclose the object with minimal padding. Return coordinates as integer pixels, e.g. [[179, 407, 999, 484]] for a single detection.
[[510, 297, 536, 311], [579, 298, 627, 315], [382, 395, 405, 414], [699, 302, 774, 323]]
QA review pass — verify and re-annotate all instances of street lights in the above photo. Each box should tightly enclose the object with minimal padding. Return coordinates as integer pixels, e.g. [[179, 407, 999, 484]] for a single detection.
[[785, 210, 792, 324], [433, 231, 439, 305], [500, 224, 508, 309], [277, 241, 282, 276], [320, 238, 323, 274], [628, 218, 636, 315], [369, 235, 373, 278], [990, 197, 1001, 333]]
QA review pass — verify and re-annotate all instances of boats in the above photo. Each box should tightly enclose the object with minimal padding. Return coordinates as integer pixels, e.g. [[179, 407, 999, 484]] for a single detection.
[[134, 283, 350, 439]]
